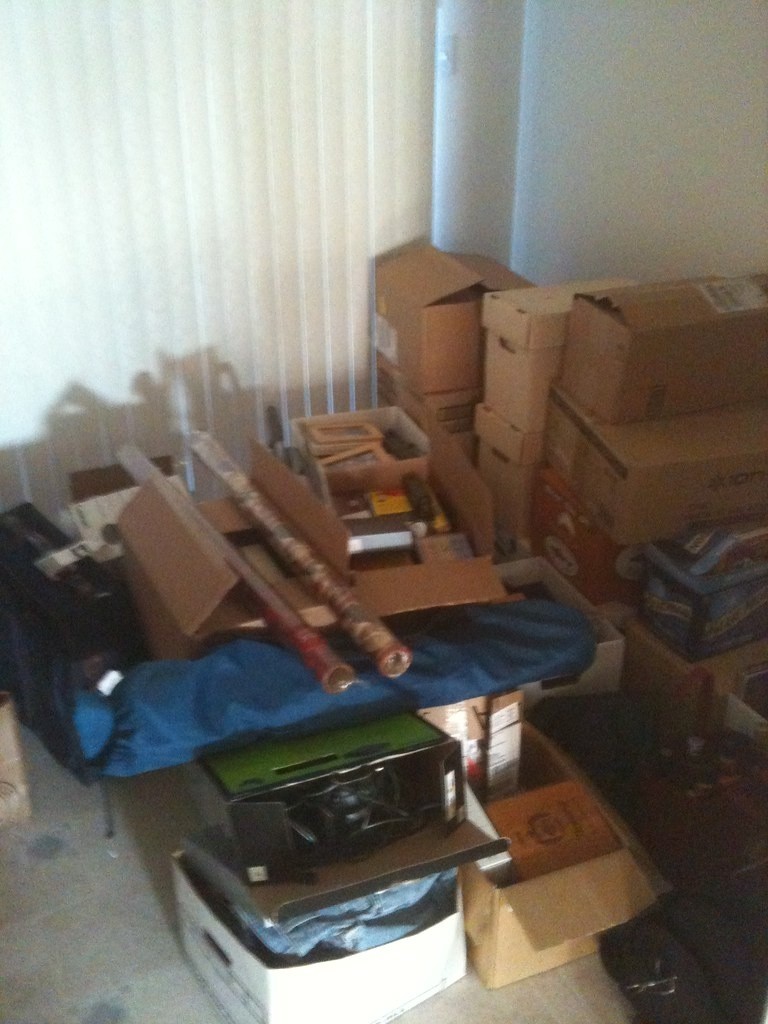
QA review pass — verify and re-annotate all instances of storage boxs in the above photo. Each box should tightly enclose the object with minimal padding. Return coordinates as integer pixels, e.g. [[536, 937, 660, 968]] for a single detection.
[[2, 234, 768, 1022]]
